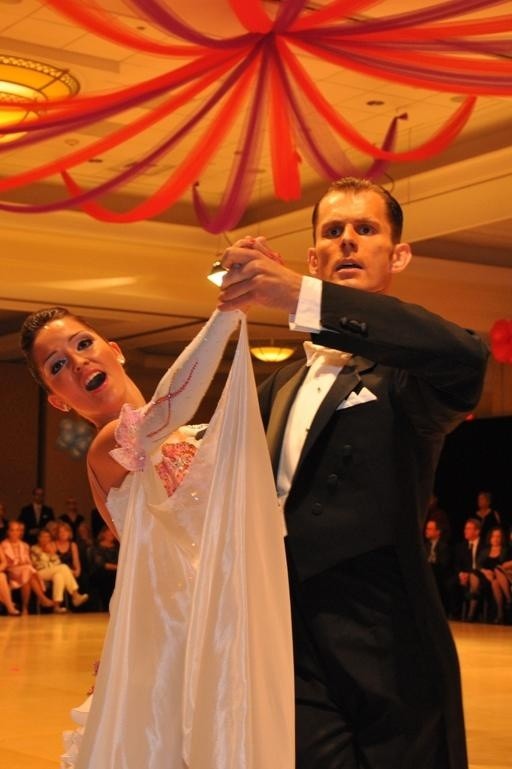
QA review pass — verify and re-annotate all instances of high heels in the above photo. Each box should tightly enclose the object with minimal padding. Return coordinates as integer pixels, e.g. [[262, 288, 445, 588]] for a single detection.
[[8, 593, 89, 615]]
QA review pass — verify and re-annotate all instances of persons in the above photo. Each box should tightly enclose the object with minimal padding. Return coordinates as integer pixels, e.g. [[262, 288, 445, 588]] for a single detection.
[[215, 176, 491, 769], [19, 235, 297, 769]]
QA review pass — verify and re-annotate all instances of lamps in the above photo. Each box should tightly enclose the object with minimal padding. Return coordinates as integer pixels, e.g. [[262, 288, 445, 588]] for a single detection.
[[251, 336, 295, 364]]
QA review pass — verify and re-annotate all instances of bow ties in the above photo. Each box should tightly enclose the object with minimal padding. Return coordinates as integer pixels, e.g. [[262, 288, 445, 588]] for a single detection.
[[302, 341, 353, 367]]
